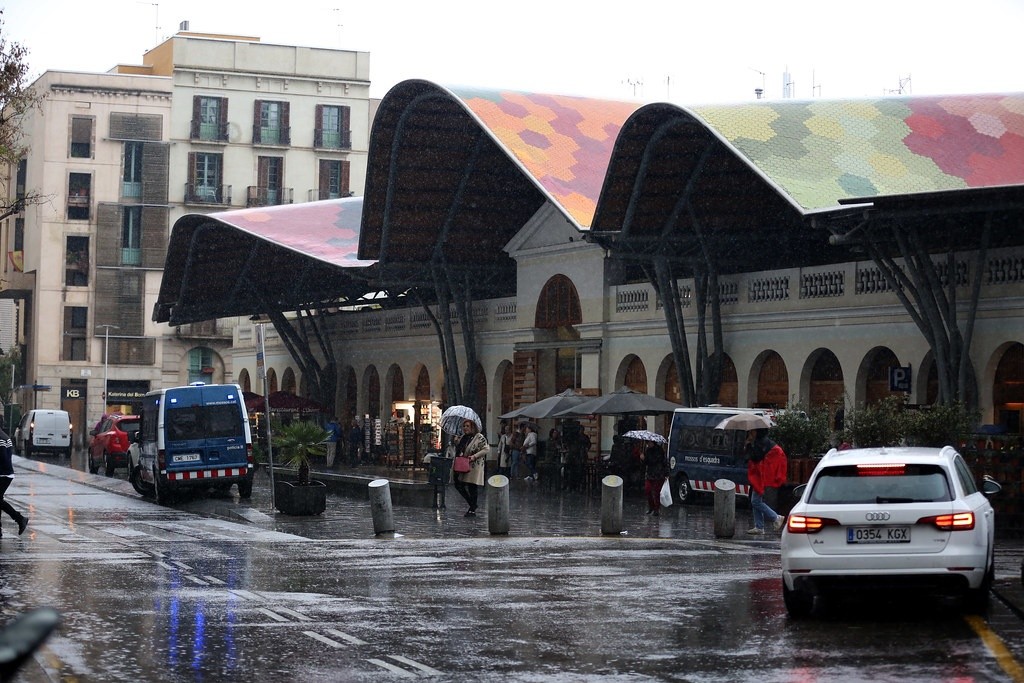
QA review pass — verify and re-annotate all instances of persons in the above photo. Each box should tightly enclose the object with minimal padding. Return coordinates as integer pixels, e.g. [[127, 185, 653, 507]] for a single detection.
[[326, 416, 339, 469], [742, 428, 788, 534], [511, 423, 525, 480], [0, 427, 29, 538], [446, 437, 455, 484], [520, 426, 540, 481], [547, 428, 570, 491], [494, 420, 508, 475], [94, 413, 107, 432], [610, 435, 635, 495], [496, 426, 514, 476], [576, 425, 591, 471], [640, 439, 670, 517], [348, 419, 362, 465], [454, 420, 490, 517]]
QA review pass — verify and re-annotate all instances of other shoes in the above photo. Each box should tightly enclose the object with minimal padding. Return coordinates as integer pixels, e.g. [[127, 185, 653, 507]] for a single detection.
[[645, 505, 658, 515], [748, 526, 764, 534], [464, 510, 476, 517], [18, 516, 29, 535], [533, 473, 538, 479], [773, 515, 784, 531], [524, 476, 533, 480]]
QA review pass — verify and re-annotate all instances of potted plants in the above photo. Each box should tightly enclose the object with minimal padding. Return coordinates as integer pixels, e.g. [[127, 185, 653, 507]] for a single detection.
[[272, 418, 336, 516], [768, 393, 835, 485]]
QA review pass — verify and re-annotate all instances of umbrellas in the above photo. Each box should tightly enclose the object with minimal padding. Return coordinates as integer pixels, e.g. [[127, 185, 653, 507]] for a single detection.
[[712, 415, 780, 445], [436, 405, 482, 437], [496, 385, 692, 481], [621, 430, 668, 452]]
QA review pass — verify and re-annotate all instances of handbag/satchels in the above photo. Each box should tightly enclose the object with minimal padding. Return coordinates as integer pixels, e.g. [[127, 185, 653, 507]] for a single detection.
[[454, 456, 472, 473], [660, 477, 673, 507], [504, 444, 510, 453]]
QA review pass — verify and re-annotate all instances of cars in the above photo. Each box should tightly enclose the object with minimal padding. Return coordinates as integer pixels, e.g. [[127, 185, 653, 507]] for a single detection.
[[87, 415, 140, 477]]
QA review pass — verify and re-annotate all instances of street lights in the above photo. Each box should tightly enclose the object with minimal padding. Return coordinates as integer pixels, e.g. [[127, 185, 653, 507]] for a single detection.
[[96, 324, 120, 414]]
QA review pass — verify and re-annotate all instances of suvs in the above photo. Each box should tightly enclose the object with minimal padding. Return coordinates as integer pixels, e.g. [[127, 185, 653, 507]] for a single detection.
[[781, 445, 1002, 620]]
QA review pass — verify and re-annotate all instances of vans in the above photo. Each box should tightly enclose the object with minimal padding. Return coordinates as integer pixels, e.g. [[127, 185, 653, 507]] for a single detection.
[[125, 382, 254, 505], [667, 403, 811, 504], [14, 410, 73, 458]]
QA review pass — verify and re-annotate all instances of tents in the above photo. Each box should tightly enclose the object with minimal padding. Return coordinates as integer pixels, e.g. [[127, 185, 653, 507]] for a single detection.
[[242, 391, 321, 426]]
